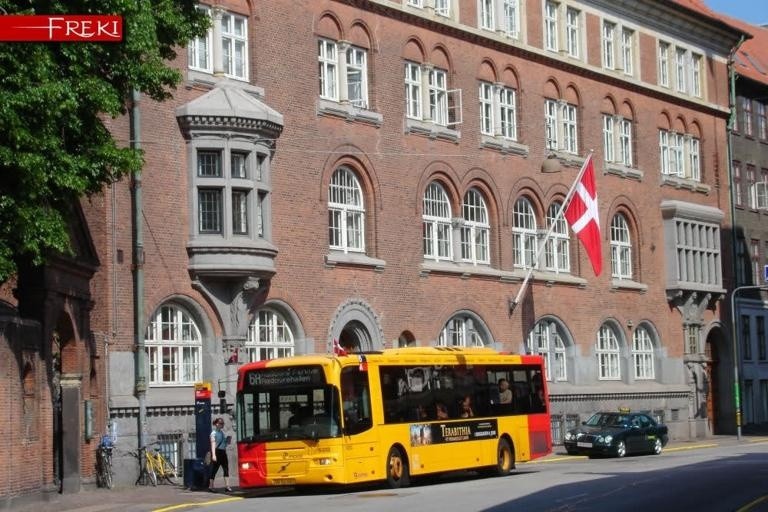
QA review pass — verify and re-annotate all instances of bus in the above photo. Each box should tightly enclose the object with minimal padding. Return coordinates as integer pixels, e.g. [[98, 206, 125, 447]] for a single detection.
[[219, 346, 552, 494]]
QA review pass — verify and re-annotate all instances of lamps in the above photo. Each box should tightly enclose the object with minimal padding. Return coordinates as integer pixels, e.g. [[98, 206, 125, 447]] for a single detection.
[[541, 154, 561, 173]]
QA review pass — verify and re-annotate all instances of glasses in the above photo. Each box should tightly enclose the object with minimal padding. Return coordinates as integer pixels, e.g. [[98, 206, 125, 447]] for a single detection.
[[217, 422, 224, 426]]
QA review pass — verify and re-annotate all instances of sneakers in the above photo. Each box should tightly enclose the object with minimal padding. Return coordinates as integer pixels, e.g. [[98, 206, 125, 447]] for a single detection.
[[208, 488, 218, 493]]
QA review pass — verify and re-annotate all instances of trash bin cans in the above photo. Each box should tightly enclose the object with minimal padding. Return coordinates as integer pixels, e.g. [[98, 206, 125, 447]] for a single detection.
[[184, 459, 211, 490]]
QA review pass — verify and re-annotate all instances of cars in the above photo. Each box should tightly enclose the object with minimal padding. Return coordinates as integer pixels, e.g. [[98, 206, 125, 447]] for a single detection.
[[564, 412, 669, 458]]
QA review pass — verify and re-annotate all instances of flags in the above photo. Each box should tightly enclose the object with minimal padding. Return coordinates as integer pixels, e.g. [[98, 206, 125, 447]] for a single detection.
[[561, 152, 603, 280]]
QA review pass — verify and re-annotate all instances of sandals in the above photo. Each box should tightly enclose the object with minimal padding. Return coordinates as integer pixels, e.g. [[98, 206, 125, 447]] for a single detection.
[[223, 486, 234, 493]]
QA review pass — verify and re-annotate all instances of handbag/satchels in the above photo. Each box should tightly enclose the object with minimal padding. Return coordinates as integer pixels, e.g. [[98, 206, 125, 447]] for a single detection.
[[203, 451, 212, 465]]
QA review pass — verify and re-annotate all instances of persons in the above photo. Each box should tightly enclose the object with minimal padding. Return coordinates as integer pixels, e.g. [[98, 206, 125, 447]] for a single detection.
[[208, 417, 233, 492], [288, 377, 544, 425]]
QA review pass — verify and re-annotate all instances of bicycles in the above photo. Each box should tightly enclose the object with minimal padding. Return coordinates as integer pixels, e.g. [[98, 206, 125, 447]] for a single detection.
[[95, 435, 115, 488], [137, 438, 180, 486]]
